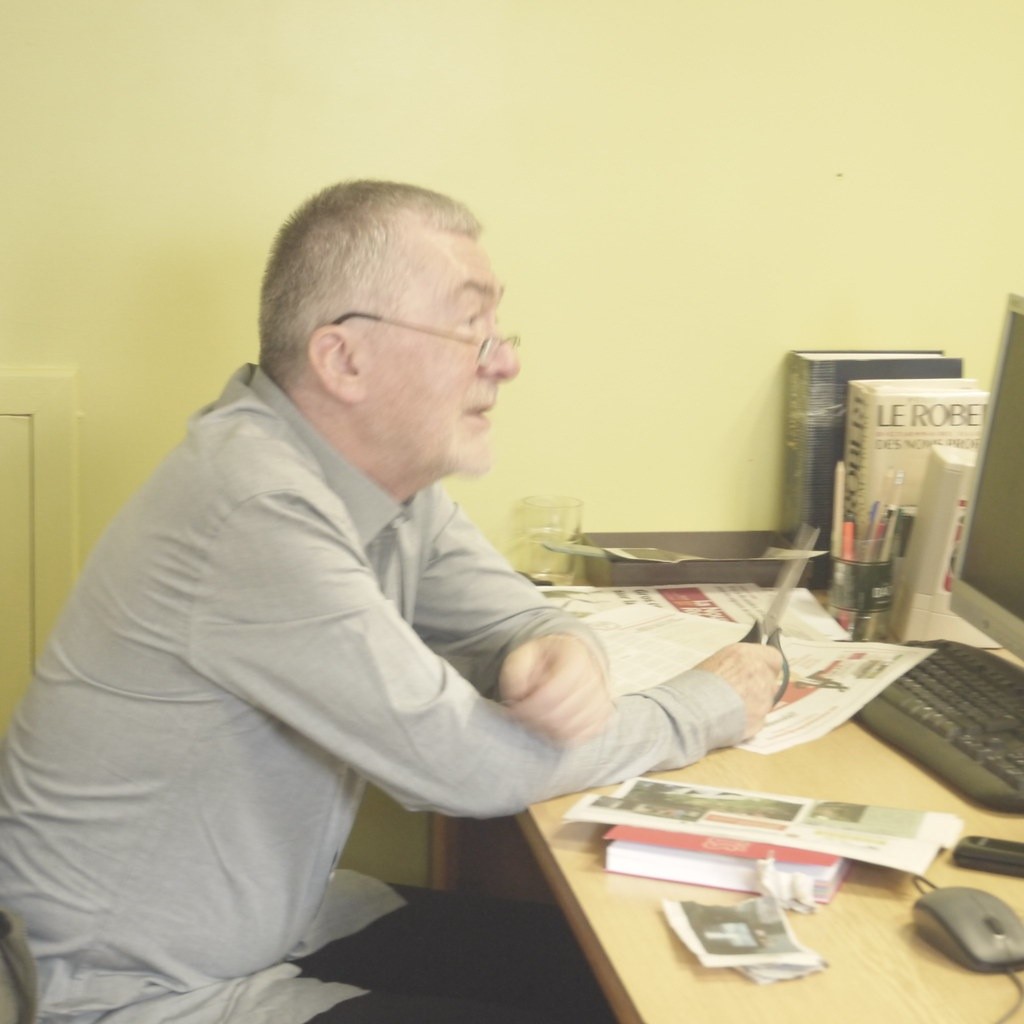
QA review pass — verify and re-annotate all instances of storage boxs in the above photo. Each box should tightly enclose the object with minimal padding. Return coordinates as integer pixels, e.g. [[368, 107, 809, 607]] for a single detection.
[[582, 529, 815, 588]]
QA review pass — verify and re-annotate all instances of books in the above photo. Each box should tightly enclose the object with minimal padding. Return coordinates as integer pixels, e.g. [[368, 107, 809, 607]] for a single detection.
[[782, 349, 963, 595], [604, 824, 850, 904], [891, 445, 1003, 648], [844, 378, 990, 563]]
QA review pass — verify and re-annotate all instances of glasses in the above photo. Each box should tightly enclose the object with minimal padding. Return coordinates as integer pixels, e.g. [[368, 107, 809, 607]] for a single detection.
[[333, 313, 520, 369]]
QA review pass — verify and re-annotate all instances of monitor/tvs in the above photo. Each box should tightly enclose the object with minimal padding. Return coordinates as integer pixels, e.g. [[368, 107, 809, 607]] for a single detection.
[[947, 290, 1024, 658]]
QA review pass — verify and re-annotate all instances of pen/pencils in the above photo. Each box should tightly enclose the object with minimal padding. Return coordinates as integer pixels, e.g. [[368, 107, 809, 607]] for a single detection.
[[831, 459, 904, 643]]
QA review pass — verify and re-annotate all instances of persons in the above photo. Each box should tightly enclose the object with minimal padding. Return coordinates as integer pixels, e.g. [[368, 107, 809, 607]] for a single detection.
[[0, 180, 785, 1024]]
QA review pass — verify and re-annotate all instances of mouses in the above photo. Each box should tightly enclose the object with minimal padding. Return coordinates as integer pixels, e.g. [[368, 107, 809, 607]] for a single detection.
[[912, 886, 1024, 974]]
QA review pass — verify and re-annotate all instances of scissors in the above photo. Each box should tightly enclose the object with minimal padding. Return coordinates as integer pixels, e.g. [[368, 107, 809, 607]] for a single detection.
[[737, 522, 822, 711]]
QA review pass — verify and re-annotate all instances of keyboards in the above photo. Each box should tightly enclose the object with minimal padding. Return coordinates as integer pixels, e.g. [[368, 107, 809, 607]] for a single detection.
[[851, 637, 1024, 815]]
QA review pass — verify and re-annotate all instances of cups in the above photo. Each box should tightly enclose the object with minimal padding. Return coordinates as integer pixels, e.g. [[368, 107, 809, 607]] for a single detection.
[[820, 555, 893, 642]]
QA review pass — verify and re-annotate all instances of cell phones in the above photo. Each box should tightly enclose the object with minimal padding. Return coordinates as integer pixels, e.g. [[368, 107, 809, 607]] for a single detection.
[[954, 836, 1024, 879]]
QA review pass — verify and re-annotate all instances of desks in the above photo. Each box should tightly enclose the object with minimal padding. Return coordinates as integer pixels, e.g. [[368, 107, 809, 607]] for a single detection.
[[428, 591, 1024, 1024]]
